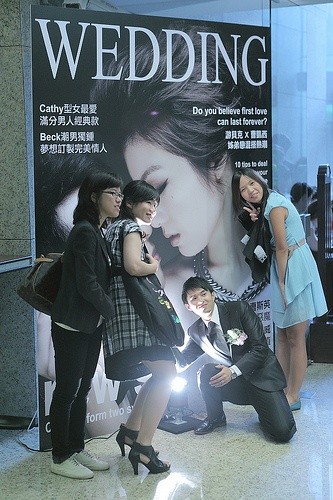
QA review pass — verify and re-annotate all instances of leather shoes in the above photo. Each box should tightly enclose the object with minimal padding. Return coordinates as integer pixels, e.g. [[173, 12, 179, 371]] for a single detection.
[[194, 412, 226, 435]]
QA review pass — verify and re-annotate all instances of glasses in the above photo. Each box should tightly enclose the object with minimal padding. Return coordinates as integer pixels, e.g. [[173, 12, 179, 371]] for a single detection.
[[102, 190, 125, 201]]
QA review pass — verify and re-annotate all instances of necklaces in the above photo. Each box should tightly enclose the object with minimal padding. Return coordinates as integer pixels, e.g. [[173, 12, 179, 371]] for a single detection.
[[193, 250, 271, 303]]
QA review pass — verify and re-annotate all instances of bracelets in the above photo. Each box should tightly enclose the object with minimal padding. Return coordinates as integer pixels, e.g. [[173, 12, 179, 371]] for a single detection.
[[228, 367, 237, 380]]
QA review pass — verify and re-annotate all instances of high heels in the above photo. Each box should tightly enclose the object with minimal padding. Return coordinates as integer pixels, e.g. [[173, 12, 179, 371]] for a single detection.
[[128, 441, 171, 475], [116, 423, 159, 457]]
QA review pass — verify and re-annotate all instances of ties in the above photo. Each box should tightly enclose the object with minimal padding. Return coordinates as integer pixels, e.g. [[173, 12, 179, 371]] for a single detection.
[[207, 322, 217, 346]]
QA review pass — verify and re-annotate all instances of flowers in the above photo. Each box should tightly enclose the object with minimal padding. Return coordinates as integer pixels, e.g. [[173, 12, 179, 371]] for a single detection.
[[226, 328, 248, 347]]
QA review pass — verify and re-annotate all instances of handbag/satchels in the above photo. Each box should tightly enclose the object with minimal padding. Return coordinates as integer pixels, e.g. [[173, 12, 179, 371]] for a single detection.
[[119, 219, 185, 347], [16, 251, 65, 317]]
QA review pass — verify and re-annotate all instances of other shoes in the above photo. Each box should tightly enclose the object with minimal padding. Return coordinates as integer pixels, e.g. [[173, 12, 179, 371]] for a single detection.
[[290, 399, 301, 411], [74, 449, 109, 470], [51, 453, 94, 479]]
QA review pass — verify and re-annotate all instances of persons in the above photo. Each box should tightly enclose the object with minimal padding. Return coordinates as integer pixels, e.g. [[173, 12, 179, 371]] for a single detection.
[[173, 275, 297, 445], [34, 23, 275, 451], [101, 179, 178, 477], [231, 165, 329, 412], [290, 179, 333, 251], [48, 171, 124, 481]]
